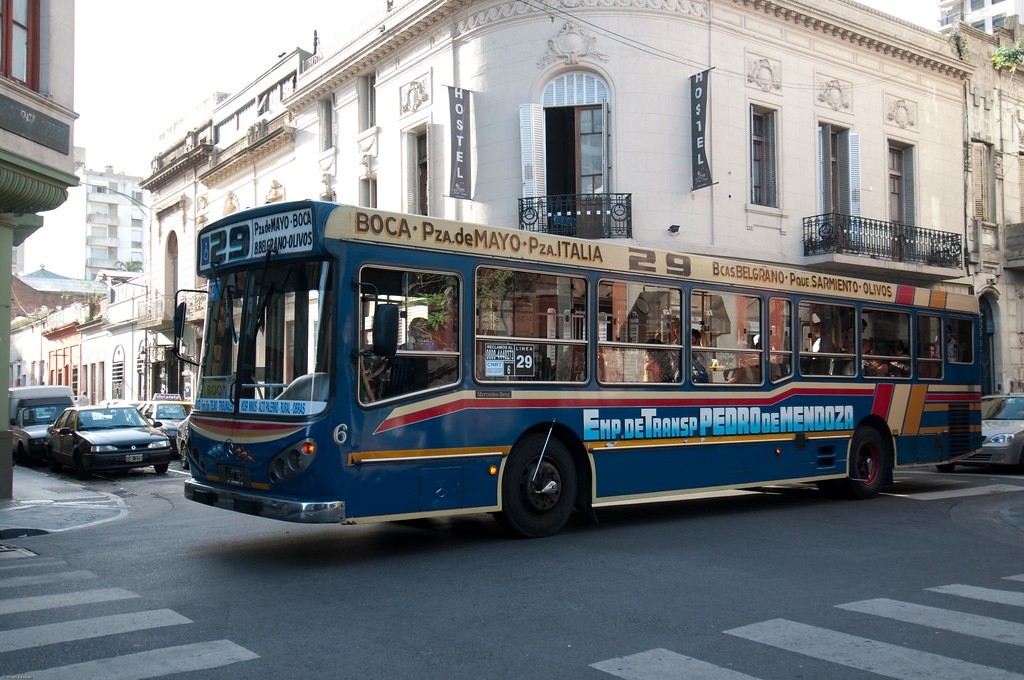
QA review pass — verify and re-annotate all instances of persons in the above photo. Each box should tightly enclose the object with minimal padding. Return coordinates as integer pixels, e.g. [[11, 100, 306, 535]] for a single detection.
[[812, 319, 963, 378], [398, 317, 436, 351], [75, 391, 89, 407], [550, 328, 709, 383]]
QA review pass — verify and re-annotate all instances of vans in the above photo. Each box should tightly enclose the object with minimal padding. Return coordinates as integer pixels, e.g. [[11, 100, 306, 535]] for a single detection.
[[9, 385, 77, 466]]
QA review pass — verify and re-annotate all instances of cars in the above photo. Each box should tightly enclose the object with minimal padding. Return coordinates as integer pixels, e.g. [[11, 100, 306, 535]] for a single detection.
[[126, 401, 188, 457], [936, 393, 1024, 475], [45, 403, 173, 480], [176, 408, 196, 471]]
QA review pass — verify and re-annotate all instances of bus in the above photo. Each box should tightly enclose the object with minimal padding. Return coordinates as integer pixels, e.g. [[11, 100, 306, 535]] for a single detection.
[[173, 198, 986, 537]]
